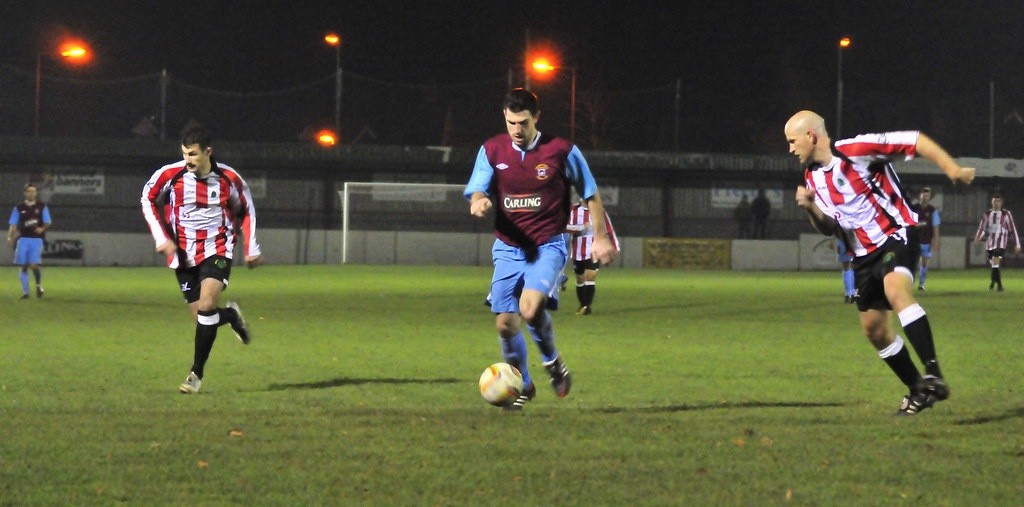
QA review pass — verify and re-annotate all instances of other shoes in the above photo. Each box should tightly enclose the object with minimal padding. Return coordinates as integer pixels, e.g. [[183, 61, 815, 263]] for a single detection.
[[575, 306, 591, 317], [36, 286, 44, 299], [19, 293, 30, 299], [846, 294, 858, 302], [918, 285, 926, 292], [989, 280, 995, 289]]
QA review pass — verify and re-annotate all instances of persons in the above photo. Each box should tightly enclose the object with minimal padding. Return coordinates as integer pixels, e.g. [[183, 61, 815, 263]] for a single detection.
[[564, 197, 620, 316], [837, 237, 854, 303], [140, 127, 261, 393], [785, 110, 975, 415], [463, 88, 615, 413], [975, 193, 1021, 291], [7, 183, 51, 299], [912, 187, 941, 290]]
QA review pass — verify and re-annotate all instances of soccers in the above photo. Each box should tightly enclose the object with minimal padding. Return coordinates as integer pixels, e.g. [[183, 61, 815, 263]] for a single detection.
[[478, 362, 524, 407]]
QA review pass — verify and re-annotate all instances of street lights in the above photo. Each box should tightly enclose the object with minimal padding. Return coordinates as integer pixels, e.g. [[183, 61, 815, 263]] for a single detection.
[[325, 35, 341, 137], [838, 38, 851, 141], [533, 58, 575, 147], [34, 44, 88, 138]]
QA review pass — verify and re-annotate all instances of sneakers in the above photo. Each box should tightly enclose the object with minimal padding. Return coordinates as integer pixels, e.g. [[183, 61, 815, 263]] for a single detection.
[[897, 394, 937, 417], [542, 355, 569, 397], [226, 303, 251, 344], [501, 382, 536, 412], [919, 373, 948, 400], [179, 371, 200, 394]]
